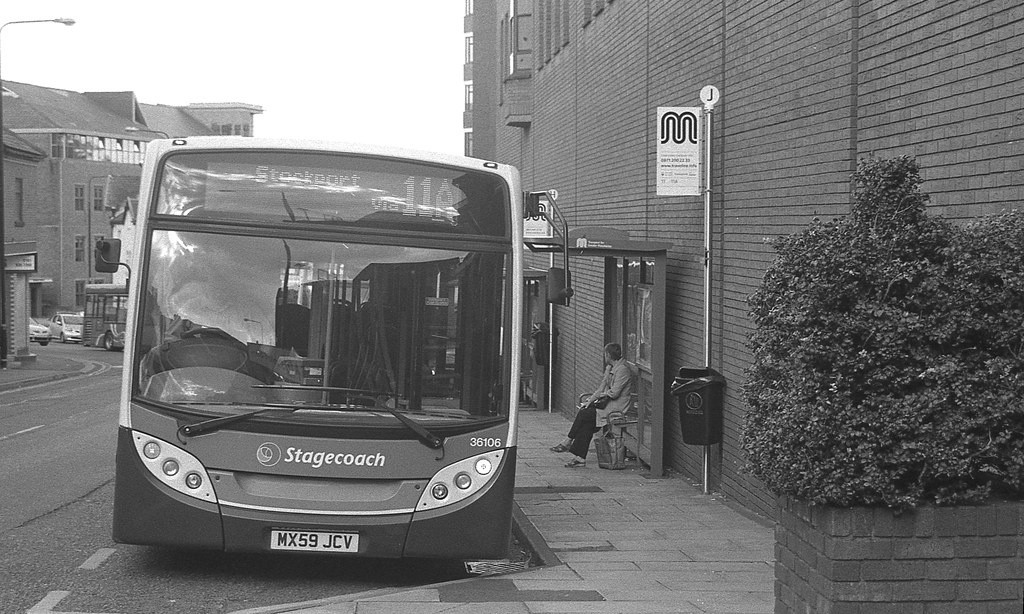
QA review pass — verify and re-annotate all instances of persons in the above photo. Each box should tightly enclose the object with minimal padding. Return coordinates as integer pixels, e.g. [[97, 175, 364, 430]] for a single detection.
[[549, 344, 632, 468], [162, 246, 275, 346]]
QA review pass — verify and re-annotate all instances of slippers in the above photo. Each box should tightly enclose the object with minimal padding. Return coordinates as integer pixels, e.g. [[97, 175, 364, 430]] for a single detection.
[[550, 444, 570, 452], [565, 460, 586, 468]]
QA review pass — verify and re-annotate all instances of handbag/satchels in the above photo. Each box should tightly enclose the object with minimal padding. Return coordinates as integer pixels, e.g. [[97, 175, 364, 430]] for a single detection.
[[593, 395, 610, 409], [595, 428, 626, 470]]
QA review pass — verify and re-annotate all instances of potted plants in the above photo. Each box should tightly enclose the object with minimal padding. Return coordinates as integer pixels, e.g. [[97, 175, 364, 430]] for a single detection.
[[736, 142, 1024, 614]]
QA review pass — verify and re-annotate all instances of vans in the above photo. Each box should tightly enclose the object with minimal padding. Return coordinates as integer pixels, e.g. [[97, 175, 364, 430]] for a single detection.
[[48, 313, 85, 343]]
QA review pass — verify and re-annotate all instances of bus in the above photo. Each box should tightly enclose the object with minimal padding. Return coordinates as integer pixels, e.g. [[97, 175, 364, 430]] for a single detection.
[[81, 283, 129, 351], [93, 132, 576, 570]]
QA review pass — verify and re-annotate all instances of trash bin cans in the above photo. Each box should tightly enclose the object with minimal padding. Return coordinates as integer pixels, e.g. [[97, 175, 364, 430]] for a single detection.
[[530, 322, 560, 365], [676, 366, 728, 445]]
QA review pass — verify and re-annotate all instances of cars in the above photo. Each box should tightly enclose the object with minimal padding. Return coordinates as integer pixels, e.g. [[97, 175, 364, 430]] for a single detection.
[[28, 317, 53, 347]]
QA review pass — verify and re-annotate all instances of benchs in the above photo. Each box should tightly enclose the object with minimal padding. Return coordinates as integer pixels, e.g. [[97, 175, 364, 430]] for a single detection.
[[569, 356, 649, 473]]
